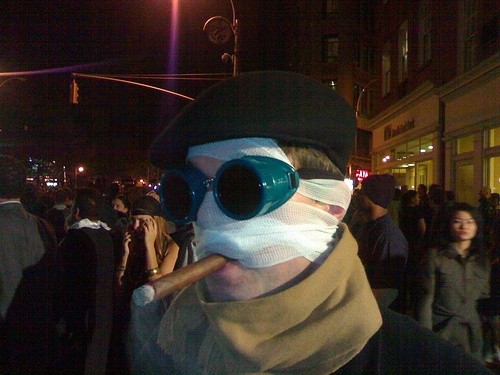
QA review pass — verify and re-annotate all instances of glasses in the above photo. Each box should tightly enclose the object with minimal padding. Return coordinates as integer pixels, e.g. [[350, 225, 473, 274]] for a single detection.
[[159, 155, 343, 225]]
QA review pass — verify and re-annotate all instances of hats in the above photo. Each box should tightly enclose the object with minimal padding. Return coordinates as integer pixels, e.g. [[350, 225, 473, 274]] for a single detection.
[[131, 196, 160, 216], [362, 174, 395, 209], [147, 71, 356, 176]]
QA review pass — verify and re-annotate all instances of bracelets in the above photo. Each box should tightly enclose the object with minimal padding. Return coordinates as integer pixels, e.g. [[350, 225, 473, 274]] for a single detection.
[[116, 266, 126, 271], [144, 268, 160, 276]]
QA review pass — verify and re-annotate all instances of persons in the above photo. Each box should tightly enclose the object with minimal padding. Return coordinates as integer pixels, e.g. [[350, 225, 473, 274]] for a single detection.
[[349, 174, 408, 314], [477, 186, 500, 362], [114, 196, 180, 295], [129, 70, 494, 375], [413, 203, 490, 366], [0, 154, 58, 375], [58, 188, 124, 375], [142, 183, 199, 271], [387, 184, 455, 282], [20, 172, 132, 242]]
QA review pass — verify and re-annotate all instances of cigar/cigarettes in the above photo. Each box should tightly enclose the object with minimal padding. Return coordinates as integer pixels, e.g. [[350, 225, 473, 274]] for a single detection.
[[132, 254, 228, 307]]
